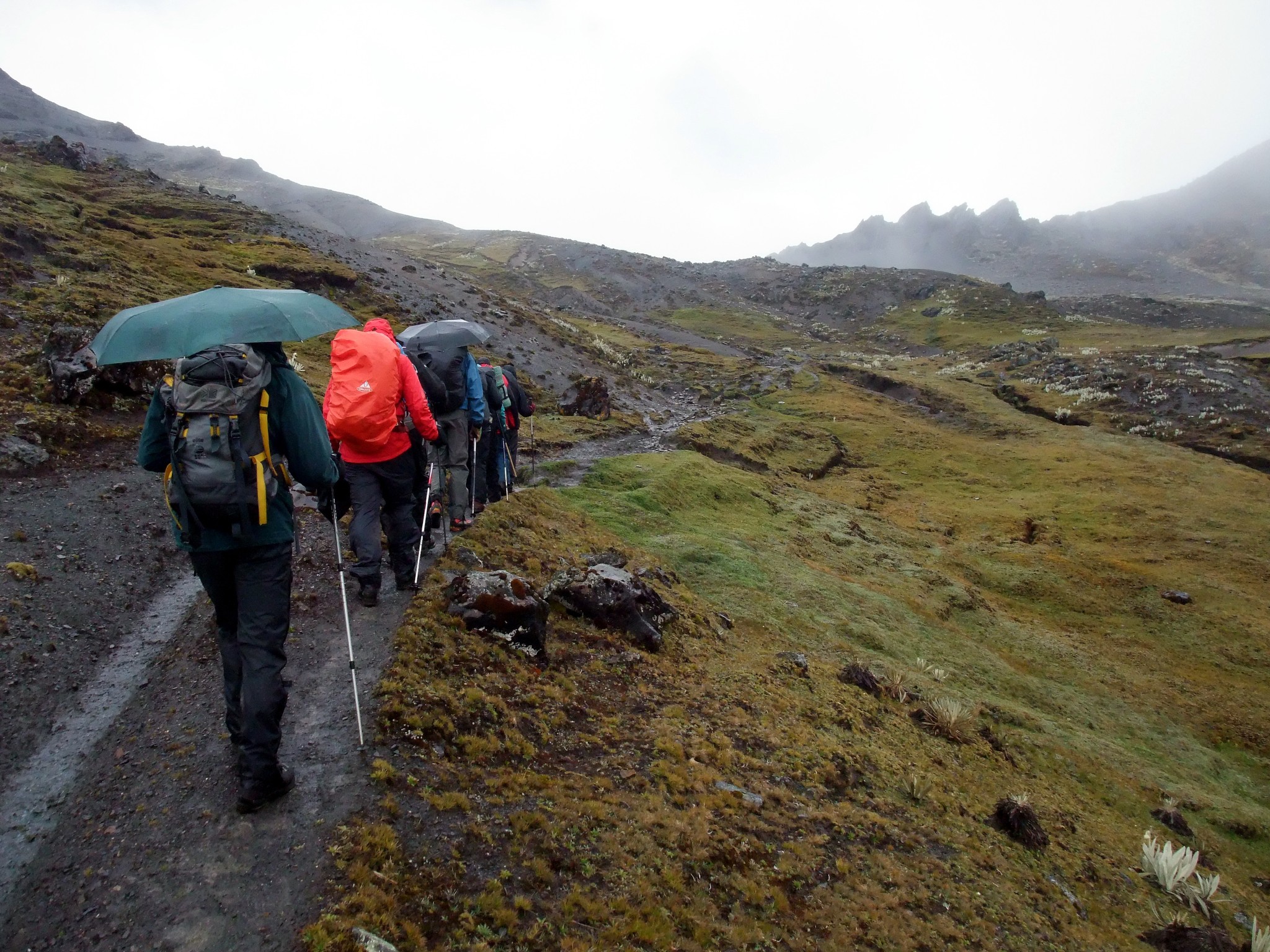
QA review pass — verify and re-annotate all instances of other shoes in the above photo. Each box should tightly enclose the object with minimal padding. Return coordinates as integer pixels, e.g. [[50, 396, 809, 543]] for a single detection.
[[511, 489, 515, 492], [474, 501, 486, 512]]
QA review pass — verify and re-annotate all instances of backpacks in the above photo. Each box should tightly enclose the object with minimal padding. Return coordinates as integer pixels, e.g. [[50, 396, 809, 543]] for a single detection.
[[476, 366, 492, 428], [163, 342, 294, 544], [402, 347, 449, 413], [493, 366, 511, 409], [325, 329, 400, 455], [421, 347, 467, 412]]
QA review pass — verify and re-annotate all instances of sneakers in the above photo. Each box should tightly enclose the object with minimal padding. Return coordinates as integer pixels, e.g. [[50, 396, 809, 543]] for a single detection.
[[235, 761, 296, 817], [415, 534, 436, 557], [232, 750, 249, 778], [430, 500, 442, 529], [450, 518, 472, 532]]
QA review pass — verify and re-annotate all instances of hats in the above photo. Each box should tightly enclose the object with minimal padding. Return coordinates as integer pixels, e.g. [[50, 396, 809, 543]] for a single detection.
[[477, 357, 491, 365]]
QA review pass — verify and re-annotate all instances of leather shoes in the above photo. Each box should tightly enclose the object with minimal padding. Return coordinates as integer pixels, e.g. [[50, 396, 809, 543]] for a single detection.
[[357, 584, 378, 606], [397, 572, 416, 590]]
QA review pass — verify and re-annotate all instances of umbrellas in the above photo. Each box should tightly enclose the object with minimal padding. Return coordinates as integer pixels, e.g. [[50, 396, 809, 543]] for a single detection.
[[89, 284, 367, 367], [393, 318, 492, 355]]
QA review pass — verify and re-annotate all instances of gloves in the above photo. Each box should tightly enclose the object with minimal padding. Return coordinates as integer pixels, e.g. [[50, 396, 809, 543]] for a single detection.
[[469, 425, 482, 443], [528, 402, 535, 414], [330, 452, 346, 484], [430, 424, 448, 450]]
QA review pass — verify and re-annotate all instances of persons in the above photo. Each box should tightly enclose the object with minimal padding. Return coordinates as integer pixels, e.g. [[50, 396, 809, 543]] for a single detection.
[[389, 333, 441, 555], [418, 344, 485, 533], [466, 351, 537, 512], [322, 317, 439, 607], [134, 343, 344, 816]]
